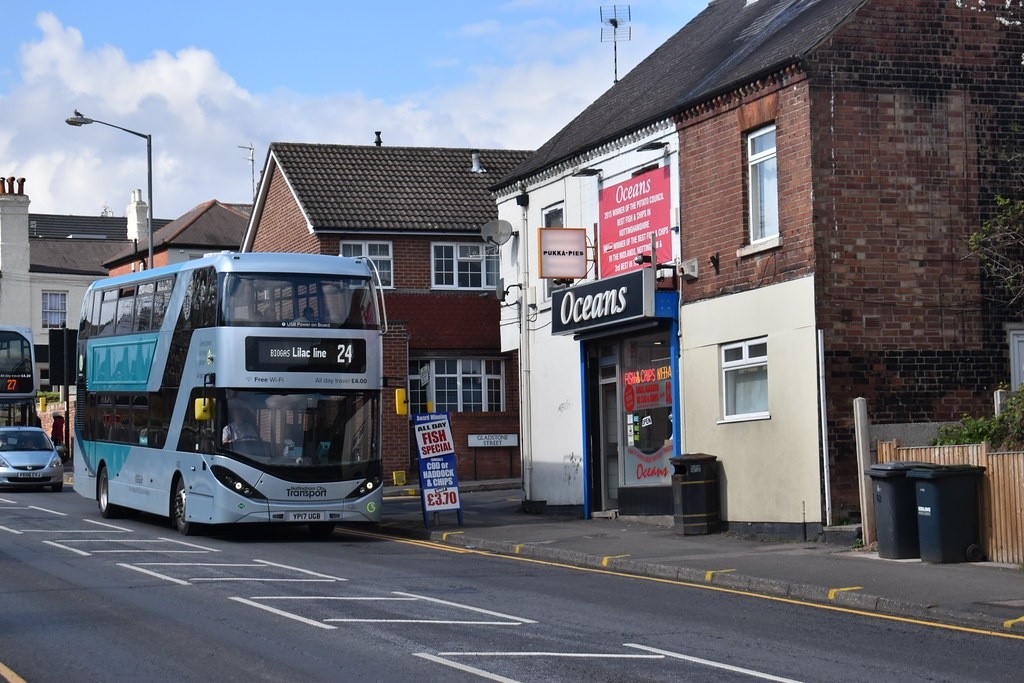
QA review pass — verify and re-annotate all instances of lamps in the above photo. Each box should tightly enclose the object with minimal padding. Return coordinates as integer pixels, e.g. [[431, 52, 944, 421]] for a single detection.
[[638, 142, 668, 151], [497, 279, 522, 301], [572, 168, 601, 178], [552, 278, 574, 285], [635, 254, 651, 265]]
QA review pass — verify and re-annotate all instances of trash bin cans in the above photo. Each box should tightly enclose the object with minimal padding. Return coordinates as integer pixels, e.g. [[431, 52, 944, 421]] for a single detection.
[[904, 464, 986, 561], [669, 453, 721, 537], [864, 462, 934, 560]]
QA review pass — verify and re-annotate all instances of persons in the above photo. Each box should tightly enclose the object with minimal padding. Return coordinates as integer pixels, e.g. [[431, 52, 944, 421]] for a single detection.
[[257, 307, 314, 326], [222, 405, 257, 443], [0, 409, 67, 465]]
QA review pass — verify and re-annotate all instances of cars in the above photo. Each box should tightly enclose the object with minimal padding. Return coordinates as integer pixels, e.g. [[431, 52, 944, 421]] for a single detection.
[[1, 426, 63, 492]]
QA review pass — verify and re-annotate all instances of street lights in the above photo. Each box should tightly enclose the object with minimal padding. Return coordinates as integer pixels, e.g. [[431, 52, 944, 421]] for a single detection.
[[65, 117, 154, 270]]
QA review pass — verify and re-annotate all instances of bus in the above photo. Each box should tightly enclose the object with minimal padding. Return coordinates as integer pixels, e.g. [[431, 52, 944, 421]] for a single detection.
[[74, 249, 384, 537], [1, 326, 37, 431]]
